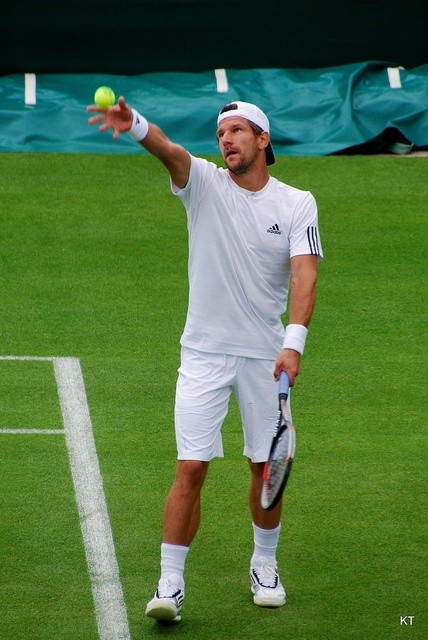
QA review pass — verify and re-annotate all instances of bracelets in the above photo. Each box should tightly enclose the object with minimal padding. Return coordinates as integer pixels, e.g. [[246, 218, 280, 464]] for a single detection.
[[122, 107, 152, 145], [281, 322, 309, 359]]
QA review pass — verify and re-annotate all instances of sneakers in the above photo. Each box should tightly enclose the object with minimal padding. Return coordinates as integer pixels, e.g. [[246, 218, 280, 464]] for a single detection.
[[248, 555, 286, 608], [143, 573, 185, 622]]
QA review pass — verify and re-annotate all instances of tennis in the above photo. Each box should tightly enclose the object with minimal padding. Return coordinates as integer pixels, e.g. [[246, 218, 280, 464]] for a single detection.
[[94, 86, 115, 108]]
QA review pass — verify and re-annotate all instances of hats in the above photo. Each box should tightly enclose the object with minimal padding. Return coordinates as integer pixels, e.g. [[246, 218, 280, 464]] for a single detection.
[[217, 100, 276, 166]]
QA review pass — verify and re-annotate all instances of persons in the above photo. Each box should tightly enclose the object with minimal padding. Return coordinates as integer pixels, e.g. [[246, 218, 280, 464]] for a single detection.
[[81, 94, 326, 625]]
